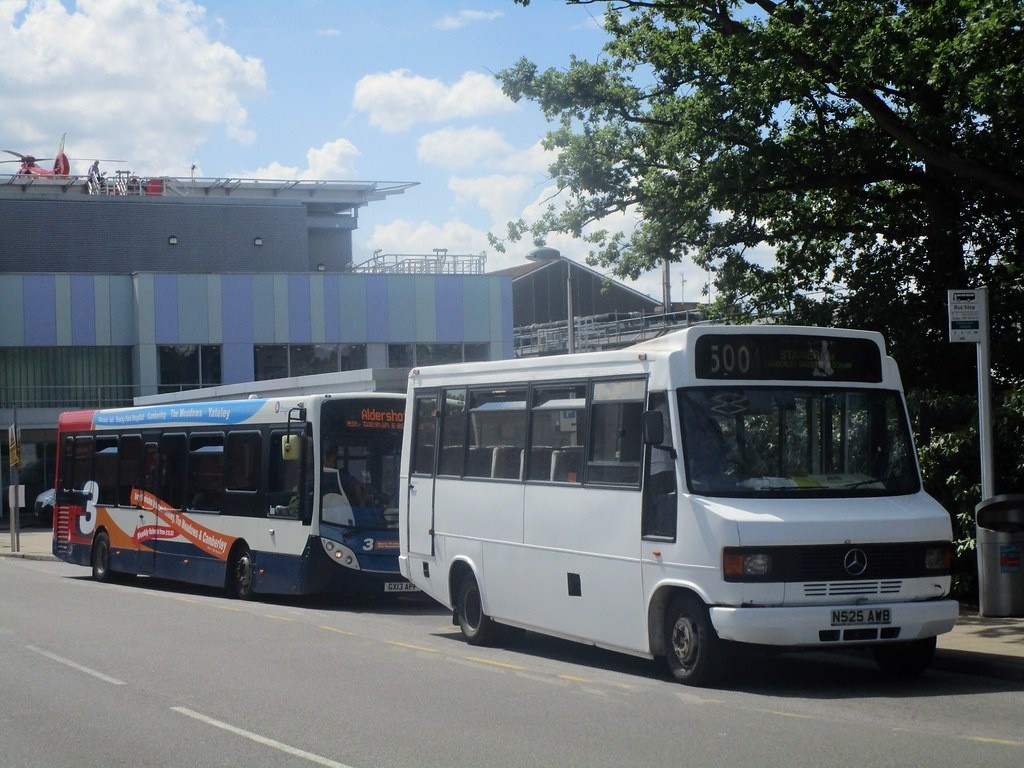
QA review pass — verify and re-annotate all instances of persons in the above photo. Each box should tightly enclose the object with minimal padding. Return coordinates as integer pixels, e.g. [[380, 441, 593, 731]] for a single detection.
[[687, 419, 741, 495], [289, 441, 362, 519], [88, 161, 100, 181]]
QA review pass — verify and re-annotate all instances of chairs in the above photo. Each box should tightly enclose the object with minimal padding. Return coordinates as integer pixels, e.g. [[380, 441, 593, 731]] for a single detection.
[[415, 443, 585, 483]]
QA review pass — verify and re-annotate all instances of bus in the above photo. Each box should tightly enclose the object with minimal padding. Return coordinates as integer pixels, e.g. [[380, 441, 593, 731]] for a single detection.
[[397, 326, 959, 688], [52, 393, 462, 602]]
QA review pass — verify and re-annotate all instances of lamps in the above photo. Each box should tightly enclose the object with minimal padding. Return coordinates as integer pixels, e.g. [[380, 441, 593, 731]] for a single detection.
[[169, 235, 178, 244], [254, 237, 263, 245], [317, 263, 325, 271]]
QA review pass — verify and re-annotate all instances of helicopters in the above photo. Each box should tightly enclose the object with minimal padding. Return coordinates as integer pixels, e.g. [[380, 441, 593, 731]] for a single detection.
[[0, 148, 127, 181]]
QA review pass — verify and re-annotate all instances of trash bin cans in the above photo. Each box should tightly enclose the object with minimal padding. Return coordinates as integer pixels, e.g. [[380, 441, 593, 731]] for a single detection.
[[974, 491, 1024, 618]]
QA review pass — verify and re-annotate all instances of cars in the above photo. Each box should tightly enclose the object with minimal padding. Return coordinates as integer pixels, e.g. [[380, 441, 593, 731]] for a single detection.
[[33, 490, 56, 522]]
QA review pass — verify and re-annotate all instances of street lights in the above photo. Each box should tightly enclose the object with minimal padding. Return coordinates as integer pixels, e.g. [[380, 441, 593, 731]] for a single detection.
[[525, 246, 575, 355]]
